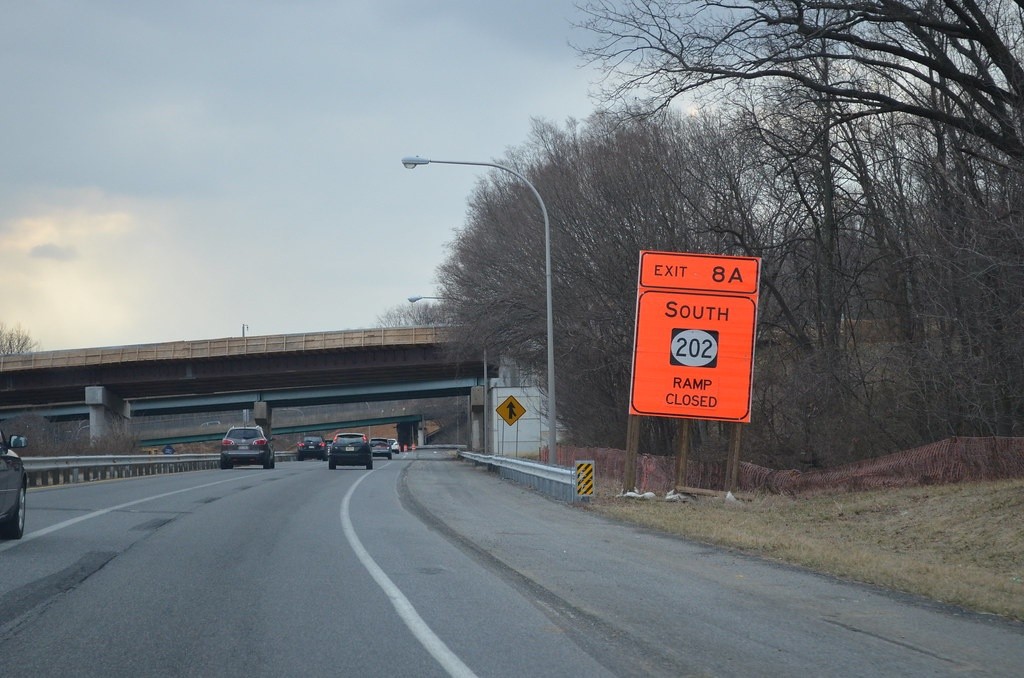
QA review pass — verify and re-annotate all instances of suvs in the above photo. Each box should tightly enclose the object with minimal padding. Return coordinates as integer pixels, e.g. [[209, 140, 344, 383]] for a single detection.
[[297, 434, 327, 461], [328, 433, 375, 470], [220, 424, 275, 470], [325, 439, 334, 454]]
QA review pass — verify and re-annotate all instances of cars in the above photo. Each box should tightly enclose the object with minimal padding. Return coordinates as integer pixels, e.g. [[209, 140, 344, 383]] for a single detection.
[[388, 439, 399, 454], [0, 428, 28, 539], [200, 420, 221, 426]]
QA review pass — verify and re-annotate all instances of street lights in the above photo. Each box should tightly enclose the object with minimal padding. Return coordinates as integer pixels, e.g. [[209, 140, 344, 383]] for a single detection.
[[402, 156, 558, 468], [406, 295, 490, 455]]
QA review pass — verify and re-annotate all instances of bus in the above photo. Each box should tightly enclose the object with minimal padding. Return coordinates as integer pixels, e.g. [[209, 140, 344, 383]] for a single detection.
[[370, 437, 392, 460]]
